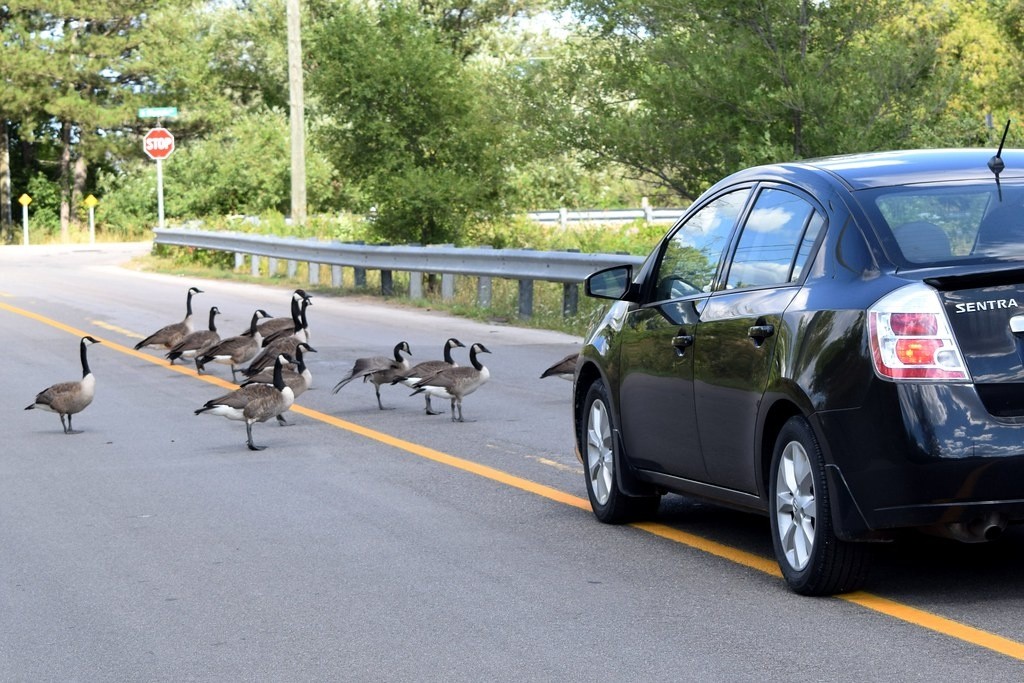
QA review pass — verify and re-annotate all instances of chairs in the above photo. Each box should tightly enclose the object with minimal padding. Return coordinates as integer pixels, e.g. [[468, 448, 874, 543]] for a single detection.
[[891, 221, 952, 261], [979, 203, 1024, 252]]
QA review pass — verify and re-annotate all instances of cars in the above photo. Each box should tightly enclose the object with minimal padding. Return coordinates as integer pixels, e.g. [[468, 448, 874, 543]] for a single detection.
[[573, 145, 1023, 598]]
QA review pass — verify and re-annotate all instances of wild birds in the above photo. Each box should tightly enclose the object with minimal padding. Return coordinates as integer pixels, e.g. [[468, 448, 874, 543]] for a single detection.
[[25, 336, 102, 434], [329, 337, 493, 423], [133, 287, 318, 453]]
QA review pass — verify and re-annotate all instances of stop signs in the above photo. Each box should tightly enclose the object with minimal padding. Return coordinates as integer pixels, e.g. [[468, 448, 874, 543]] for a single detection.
[[143, 127, 175, 160]]
